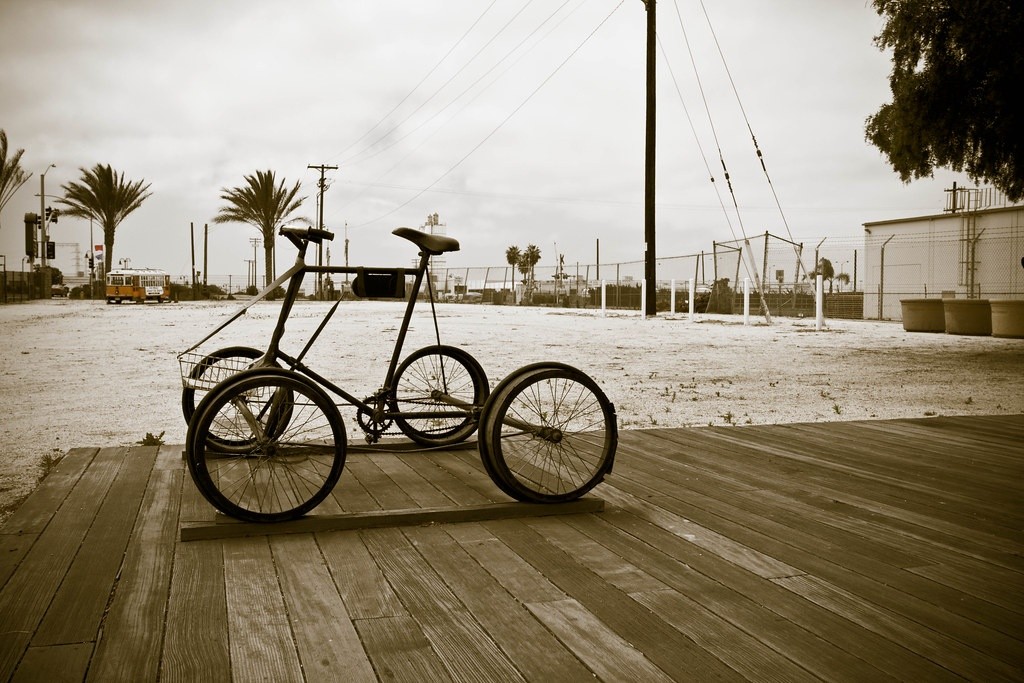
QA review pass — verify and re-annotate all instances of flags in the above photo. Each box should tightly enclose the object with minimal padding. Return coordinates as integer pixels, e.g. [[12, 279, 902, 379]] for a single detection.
[[96, 254, 102, 260], [95, 245, 103, 251]]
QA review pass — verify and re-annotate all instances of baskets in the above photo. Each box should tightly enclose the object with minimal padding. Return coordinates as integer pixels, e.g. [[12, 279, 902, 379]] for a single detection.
[[177, 350, 265, 398]]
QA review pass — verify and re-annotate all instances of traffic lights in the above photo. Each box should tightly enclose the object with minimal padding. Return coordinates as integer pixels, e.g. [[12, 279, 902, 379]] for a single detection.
[[90, 273, 95, 278], [46, 207, 51, 220], [52, 209, 57, 224], [89, 258, 92, 268], [119, 257, 132, 269]]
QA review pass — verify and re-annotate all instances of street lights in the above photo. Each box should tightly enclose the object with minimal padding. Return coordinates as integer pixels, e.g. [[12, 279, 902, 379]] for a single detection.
[[21, 256, 30, 272], [38, 216, 42, 228], [35, 193, 93, 297], [0, 255, 8, 303], [84, 250, 94, 298], [228, 274, 232, 294], [40, 162, 56, 265]]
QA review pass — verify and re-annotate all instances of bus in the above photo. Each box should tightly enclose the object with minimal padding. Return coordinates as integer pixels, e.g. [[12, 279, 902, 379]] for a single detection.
[[106, 268, 171, 304]]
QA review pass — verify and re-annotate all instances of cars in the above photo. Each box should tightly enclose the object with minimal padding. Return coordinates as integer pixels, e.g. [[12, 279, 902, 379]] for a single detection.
[[52, 285, 67, 297]]
